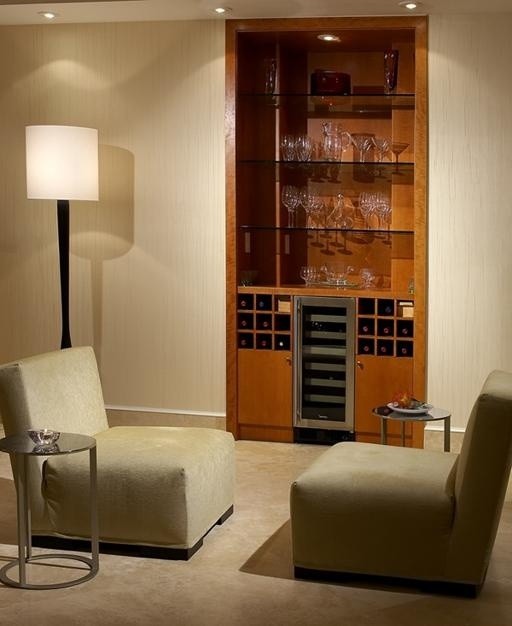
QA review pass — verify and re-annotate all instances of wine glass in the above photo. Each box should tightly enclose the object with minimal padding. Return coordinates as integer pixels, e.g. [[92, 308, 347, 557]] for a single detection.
[[299, 264, 379, 289], [282, 120, 410, 228]]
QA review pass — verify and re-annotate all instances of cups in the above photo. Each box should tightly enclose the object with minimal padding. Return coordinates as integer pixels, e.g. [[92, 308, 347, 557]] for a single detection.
[[239, 269, 261, 286], [382, 49, 398, 94], [408, 280, 415, 294]]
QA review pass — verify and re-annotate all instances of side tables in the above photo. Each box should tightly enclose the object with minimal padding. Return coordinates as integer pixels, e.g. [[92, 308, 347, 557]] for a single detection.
[[373, 407, 452, 457], [0, 432, 99, 591]]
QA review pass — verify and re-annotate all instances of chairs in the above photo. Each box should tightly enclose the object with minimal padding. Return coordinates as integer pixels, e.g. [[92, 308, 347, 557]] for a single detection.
[[1, 346, 235, 561], [291, 369, 510, 598]]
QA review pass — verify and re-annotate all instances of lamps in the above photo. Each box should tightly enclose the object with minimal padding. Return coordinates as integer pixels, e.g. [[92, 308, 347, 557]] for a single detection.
[[25, 124, 99, 348]]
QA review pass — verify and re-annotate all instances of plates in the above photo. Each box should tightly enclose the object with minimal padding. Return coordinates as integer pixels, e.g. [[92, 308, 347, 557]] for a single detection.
[[387, 400, 435, 414]]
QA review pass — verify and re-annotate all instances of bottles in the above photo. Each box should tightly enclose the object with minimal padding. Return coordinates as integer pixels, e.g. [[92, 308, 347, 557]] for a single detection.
[[265, 59, 276, 92]]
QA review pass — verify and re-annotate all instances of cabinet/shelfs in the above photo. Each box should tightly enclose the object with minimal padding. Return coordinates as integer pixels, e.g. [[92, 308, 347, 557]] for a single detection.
[[226, 17, 428, 447]]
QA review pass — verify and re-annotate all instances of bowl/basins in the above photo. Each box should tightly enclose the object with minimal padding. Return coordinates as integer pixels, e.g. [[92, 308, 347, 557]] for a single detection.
[[28, 428, 60, 445], [32, 443, 59, 454]]
